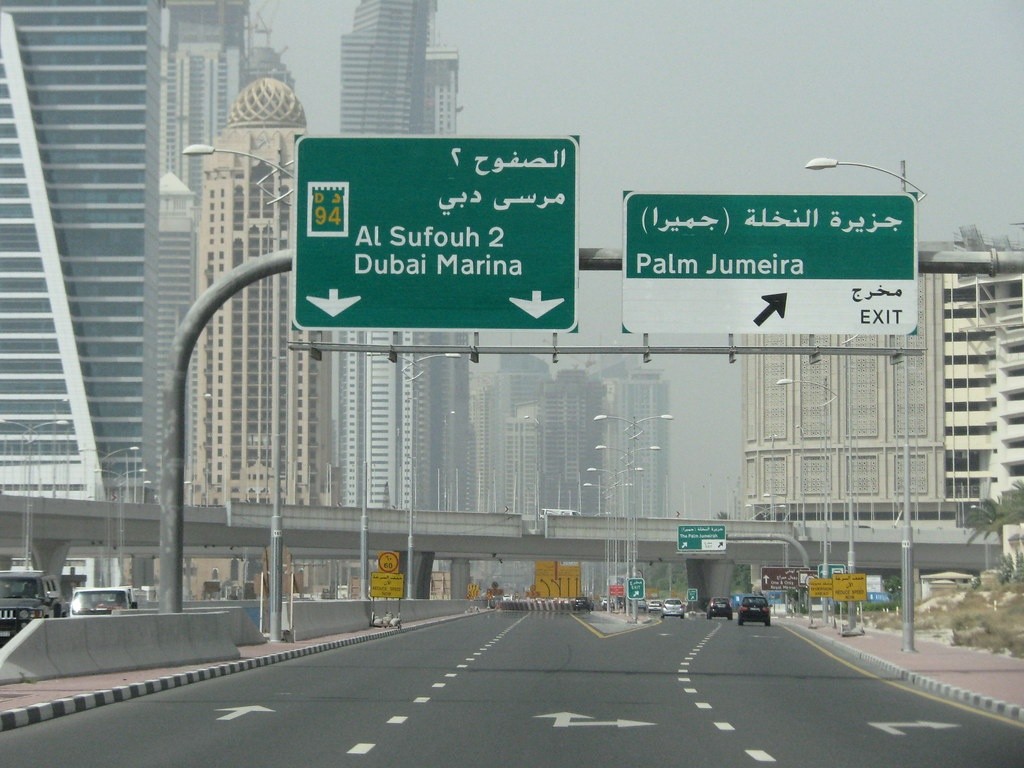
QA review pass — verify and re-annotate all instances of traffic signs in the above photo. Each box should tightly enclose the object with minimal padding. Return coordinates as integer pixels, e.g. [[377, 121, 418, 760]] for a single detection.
[[761, 566, 810, 591], [622, 190, 918, 335], [627, 578, 645, 600], [677, 525, 726, 550], [292, 134, 579, 334], [687, 589, 697, 601]]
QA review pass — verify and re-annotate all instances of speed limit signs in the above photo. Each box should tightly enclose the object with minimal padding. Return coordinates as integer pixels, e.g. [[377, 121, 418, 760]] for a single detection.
[[378, 552, 398, 573]]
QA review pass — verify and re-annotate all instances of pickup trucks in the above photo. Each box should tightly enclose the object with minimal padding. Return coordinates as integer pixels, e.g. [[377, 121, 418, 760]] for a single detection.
[[737, 596, 774, 625]]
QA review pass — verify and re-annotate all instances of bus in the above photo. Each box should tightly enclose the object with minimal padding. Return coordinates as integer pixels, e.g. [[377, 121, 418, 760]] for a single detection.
[[540, 509, 582, 519]]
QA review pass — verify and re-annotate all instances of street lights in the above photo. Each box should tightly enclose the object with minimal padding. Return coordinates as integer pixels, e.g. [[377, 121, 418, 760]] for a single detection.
[[583, 414, 674, 623], [744, 157, 926, 654], [0, 145, 462, 599]]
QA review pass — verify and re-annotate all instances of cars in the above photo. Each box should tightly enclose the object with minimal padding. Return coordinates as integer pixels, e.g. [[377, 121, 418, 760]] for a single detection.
[[648, 600, 662, 613], [638, 600, 647, 611], [0, 570, 137, 646], [659, 599, 685, 619], [572, 597, 594, 611], [706, 597, 733, 620], [600, 598, 614, 610], [615, 596, 625, 609]]
